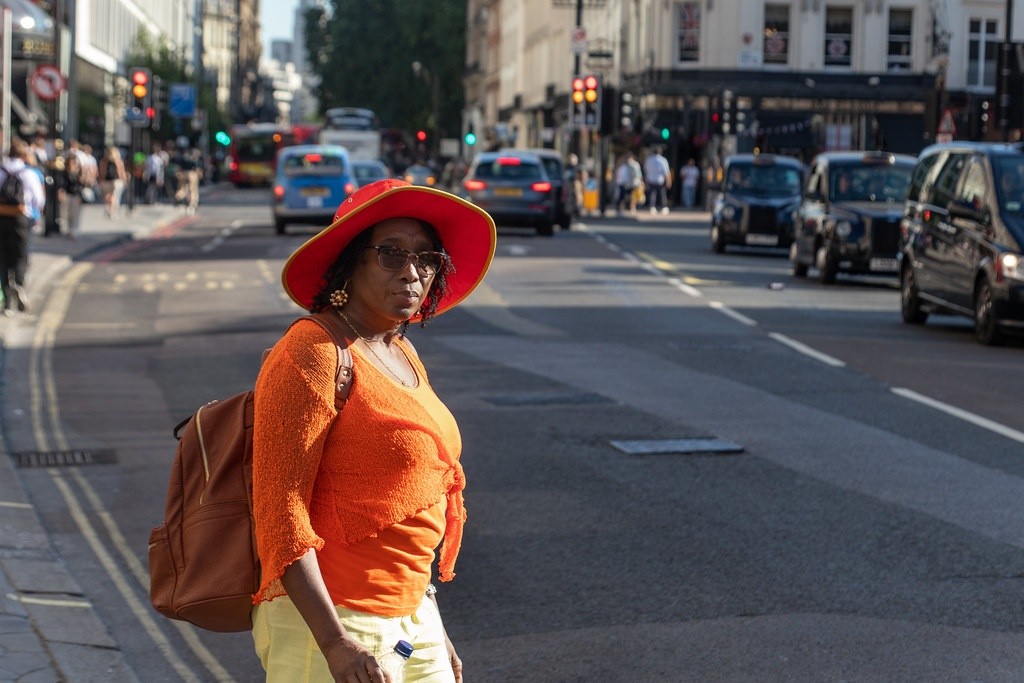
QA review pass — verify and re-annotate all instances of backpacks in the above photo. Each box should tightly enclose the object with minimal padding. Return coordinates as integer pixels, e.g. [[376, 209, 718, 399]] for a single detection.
[[1, 165, 28, 205], [104, 160, 119, 180], [147, 313, 354, 632]]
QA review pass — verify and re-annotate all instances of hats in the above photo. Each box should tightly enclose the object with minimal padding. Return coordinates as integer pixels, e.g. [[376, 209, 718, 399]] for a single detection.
[[282, 178, 498, 323]]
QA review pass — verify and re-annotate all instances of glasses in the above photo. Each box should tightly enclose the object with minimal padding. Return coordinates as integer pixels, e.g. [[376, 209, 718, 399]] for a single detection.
[[358, 245, 446, 275]]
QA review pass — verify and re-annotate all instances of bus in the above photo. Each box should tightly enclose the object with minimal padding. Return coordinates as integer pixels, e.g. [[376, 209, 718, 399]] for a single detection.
[[228, 123, 285, 188], [316, 129, 385, 160]]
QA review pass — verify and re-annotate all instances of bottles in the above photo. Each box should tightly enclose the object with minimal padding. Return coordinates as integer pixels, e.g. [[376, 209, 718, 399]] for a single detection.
[[374, 640, 414, 683]]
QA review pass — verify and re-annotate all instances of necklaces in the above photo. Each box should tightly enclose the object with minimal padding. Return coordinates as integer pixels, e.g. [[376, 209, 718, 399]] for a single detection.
[[337, 310, 418, 387]]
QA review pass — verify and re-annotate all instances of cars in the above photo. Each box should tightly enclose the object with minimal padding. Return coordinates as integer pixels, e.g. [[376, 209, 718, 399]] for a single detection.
[[270, 145, 361, 235], [457, 152, 557, 236], [500, 147, 576, 229], [711, 154, 807, 256], [788, 147, 919, 285], [352, 159, 393, 189], [404, 164, 437, 186]]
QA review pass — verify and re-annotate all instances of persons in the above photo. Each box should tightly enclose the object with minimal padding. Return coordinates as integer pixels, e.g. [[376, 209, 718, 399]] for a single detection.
[[253, 179, 497, 683], [384, 139, 468, 196], [1000, 164, 1021, 199], [739, 173, 754, 188], [1006, 123, 1022, 142], [835, 174, 854, 199], [0, 138, 227, 316], [564, 143, 723, 219]]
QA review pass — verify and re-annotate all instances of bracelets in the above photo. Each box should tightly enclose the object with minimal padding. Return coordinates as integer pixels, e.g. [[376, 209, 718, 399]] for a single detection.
[[425, 584, 437, 597]]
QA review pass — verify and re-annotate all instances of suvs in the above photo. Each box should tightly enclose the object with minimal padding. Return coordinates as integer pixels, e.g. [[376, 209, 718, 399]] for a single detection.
[[895, 138, 1024, 347]]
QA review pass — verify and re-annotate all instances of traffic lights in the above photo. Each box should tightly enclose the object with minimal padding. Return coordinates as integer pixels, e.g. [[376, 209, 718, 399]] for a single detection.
[[718, 87, 738, 136], [710, 108, 720, 135], [582, 72, 603, 128], [617, 89, 636, 131], [567, 76, 586, 131], [735, 97, 752, 134], [124, 66, 152, 128], [414, 127, 431, 146]]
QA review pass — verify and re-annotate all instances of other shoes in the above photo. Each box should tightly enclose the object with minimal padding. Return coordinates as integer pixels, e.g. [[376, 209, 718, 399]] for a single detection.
[[1, 300, 12, 316], [11, 286, 26, 312]]
